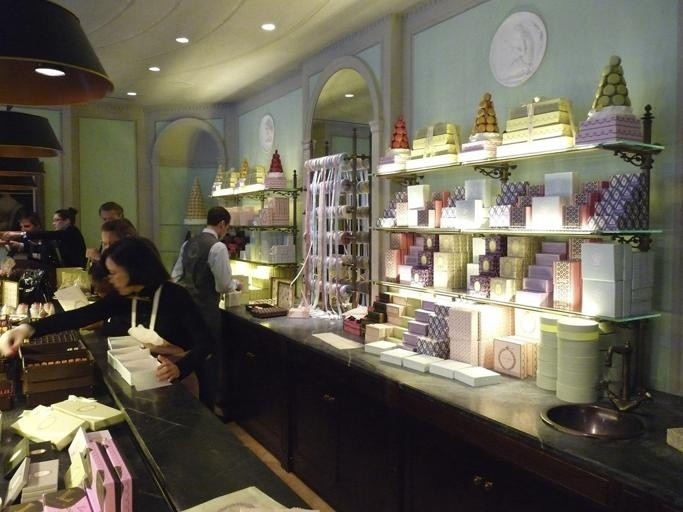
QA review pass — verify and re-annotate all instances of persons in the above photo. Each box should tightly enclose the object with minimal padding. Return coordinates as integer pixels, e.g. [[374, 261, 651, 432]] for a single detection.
[[86, 202, 162, 275], [88, 219, 171, 300], [0, 208, 86, 268], [0, 236, 220, 414], [170, 206, 242, 349], [0, 211, 59, 291]]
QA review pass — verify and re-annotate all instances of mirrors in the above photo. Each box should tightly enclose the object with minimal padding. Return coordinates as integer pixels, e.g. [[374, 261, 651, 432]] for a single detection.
[[310, 67, 371, 312]]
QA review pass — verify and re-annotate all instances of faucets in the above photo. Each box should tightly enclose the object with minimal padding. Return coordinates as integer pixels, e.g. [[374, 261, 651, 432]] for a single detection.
[[606, 341, 632, 400]]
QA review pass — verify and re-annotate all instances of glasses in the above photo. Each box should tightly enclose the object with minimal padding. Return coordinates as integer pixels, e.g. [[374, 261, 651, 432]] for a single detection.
[[17, 225, 32, 229], [103, 269, 125, 277], [51, 217, 64, 223]]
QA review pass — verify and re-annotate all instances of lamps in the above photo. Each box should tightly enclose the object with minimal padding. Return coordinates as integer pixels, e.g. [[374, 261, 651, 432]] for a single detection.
[[0, 176, 39, 193], [0, 1, 115, 110], [0, 111, 65, 159], [0, 159, 46, 176]]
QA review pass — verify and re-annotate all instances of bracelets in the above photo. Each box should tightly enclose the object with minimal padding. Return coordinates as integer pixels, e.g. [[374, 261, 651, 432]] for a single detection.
[[21, 231, 27, 239]]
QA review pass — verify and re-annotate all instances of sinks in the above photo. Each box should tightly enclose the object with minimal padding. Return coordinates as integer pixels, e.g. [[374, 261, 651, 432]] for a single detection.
[[540, 401, 649, 440]]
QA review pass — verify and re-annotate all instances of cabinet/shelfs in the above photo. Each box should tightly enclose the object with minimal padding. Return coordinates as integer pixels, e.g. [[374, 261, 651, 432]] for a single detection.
[[403, 389, 683, 511], [324, 130, 371, 306], [291, 344, 402, 512], [207, 128, 371, 313], [220, 311, 290, 472], [371, 103, 666, 394], [158, 224, 218, 274]]
